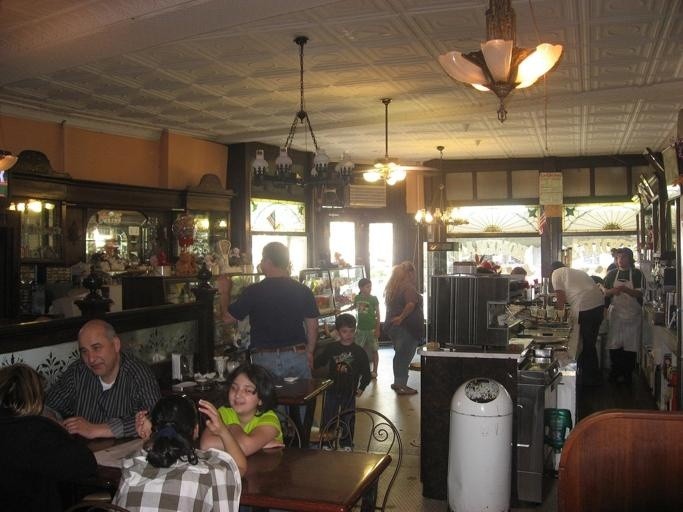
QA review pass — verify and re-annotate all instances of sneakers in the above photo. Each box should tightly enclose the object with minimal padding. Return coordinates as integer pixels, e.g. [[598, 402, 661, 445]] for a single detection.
[[391, 384, 417, 394]]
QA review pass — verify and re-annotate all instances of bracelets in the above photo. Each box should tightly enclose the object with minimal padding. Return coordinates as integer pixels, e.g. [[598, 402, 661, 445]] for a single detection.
[[306, 348, 314, 357]]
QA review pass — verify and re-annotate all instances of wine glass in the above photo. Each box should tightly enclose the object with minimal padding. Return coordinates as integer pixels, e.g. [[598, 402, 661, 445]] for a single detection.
[[213, 356, 230, 382]]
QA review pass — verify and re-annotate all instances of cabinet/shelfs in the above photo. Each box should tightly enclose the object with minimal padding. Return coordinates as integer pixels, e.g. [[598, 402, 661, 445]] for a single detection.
[[167, 276, 252, 357], [296, 265, 363, 361], [633, 195, 683, 413]]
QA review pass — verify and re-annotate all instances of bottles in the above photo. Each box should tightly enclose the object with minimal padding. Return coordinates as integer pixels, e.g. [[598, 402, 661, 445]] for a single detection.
[[542, 271, 550, 294]]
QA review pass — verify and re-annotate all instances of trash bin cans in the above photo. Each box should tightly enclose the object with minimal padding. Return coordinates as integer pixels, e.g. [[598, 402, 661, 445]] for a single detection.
[[447, 379, 513, 511]]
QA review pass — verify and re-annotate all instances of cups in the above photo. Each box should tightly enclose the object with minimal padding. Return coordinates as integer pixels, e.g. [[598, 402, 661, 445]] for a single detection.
[[227, 361, 240, 374]]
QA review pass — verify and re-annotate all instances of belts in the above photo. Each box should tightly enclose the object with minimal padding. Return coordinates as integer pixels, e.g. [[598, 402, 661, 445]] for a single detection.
[[256, 346, 306, 353]]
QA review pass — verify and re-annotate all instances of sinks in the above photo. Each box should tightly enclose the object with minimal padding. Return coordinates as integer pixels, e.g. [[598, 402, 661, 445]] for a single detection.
[[532, 322, 571, 329]]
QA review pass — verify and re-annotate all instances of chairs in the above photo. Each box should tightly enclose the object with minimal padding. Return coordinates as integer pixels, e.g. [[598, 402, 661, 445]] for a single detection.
[[319, 407, 405, 512], [275, 407, 303, 448]]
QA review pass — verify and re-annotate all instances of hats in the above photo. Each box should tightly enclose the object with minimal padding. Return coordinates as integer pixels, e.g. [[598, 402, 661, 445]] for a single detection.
[[616, 247, 633, 256]]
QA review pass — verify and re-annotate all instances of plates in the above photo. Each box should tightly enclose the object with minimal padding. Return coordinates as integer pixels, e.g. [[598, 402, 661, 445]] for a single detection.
[[283, 377, 298, 381]]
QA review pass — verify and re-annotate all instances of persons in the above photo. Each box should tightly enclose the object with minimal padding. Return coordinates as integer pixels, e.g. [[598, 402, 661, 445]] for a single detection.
[[45, 317, 163, 440], [312, 314, 372, 453], [217, 245, 320, 449], [108, 392, 248, 512], [509, 260, 606, 380], [199, 363, 287, 458], [384, 261, 423, 394], [0, 362, 101, 512], [604, 247, 646, 387], [349, 277, 382, 380]]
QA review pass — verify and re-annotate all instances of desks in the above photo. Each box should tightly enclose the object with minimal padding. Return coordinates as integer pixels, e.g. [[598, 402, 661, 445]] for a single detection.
[[161, 374, 334, 429], [80, 440, 392, 511]]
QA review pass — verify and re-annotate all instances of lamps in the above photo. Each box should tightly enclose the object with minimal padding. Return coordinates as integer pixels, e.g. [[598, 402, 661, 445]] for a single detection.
[[434, 1, 564, 121], [351, 97, 439, 190], [414, 145, 469, 225], [244, 37, 354, 206]]
